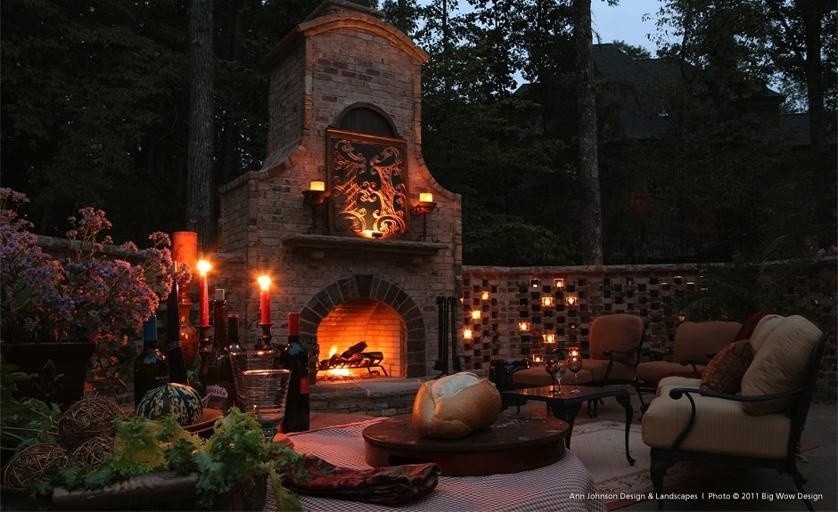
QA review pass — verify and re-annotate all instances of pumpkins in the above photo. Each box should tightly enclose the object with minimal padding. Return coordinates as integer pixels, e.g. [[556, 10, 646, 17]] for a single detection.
[[137, 381, 203, 428]]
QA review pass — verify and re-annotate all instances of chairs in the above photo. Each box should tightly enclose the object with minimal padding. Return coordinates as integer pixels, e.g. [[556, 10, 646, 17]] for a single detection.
[[513, 313, 823, 511]]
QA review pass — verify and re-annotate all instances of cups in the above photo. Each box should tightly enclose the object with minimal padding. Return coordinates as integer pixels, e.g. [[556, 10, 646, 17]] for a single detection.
[[228, 344, 276, 408]]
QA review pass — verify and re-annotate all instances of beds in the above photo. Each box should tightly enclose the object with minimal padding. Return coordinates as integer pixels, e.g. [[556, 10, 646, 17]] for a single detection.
[[501, 384, 637, 468]]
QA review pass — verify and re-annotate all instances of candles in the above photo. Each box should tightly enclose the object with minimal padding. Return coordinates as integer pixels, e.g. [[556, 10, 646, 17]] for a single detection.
[[226, 313, 239, 341], [171, 230, 197, 274], [258, 275, 271, 325], [214, 287, 224, 301], [197, 256, 212, 327], [287, 311, 299, 337]]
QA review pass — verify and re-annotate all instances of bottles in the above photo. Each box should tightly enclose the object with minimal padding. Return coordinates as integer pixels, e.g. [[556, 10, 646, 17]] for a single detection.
[[133, 309, 172, 404], [177, 301, 243, 415], [280, 311, 310, 438]]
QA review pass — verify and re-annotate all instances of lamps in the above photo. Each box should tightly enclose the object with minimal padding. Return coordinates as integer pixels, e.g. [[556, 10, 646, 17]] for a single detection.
[[300, 180, 329, 235], [412, 192, 442, 243]]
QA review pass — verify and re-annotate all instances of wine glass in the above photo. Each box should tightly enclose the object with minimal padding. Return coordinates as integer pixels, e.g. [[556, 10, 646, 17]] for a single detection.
[[543, 342, 583, 395], [241, 368, 290, 444]]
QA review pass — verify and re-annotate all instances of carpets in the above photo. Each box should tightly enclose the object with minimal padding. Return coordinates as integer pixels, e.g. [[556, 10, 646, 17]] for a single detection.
[[564, 419, 821, 512]]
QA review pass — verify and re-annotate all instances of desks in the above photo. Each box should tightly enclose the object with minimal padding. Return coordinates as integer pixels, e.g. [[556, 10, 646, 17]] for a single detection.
[[264, 416, 609, 512], [48, 457, 272, 511]]
